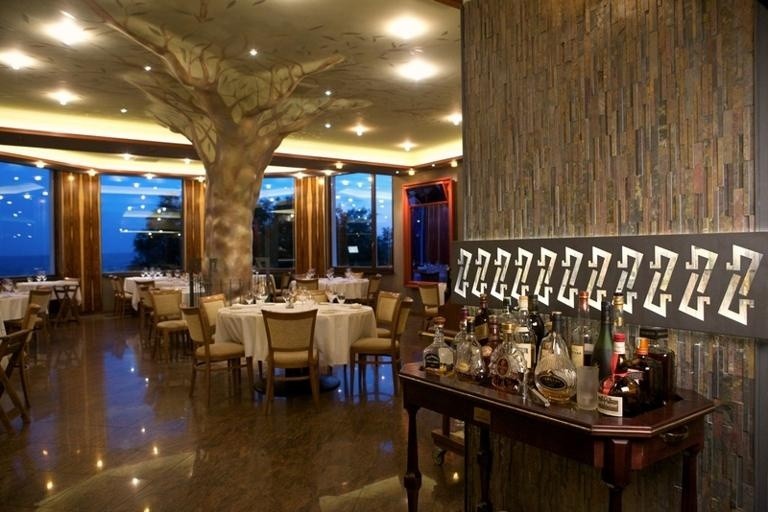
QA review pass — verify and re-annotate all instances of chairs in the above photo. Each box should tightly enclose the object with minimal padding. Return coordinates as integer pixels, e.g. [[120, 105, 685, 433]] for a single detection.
[[1, 272, 85, 402], [259, 308, 321, 403], [416, 282, 440, 334], [258, 271, 381, 302], [177, 292, 253, 403], [348, 289, 414, 402], [108, 273, 192, 367]]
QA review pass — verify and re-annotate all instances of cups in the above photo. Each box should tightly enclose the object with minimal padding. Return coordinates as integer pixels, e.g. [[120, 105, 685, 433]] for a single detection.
[[27, 278, 32, 283]]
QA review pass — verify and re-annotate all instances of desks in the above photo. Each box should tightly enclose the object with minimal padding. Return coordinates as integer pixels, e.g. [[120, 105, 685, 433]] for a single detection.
[[290, 276, 369, 301], [1, 292, 30, 322], [216, 302, 378, 366], [123, 275, 190, 297], [15, 281, 83, 306], [132, 281, 205, 311], [398, 361, 715, 511]]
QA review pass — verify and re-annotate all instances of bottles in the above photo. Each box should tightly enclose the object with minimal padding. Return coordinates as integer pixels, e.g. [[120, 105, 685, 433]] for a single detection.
[[421, 288, 677, 418]]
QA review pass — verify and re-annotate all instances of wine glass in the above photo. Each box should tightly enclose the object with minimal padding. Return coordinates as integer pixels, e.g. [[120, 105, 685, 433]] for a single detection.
[[2, 278, 14, 296], [244, 285, 347, 309], [255, 263, 260, 276], [36, 271, 47, 284], [140, 267, 190, 283], [305, 267, 356, 281]]
